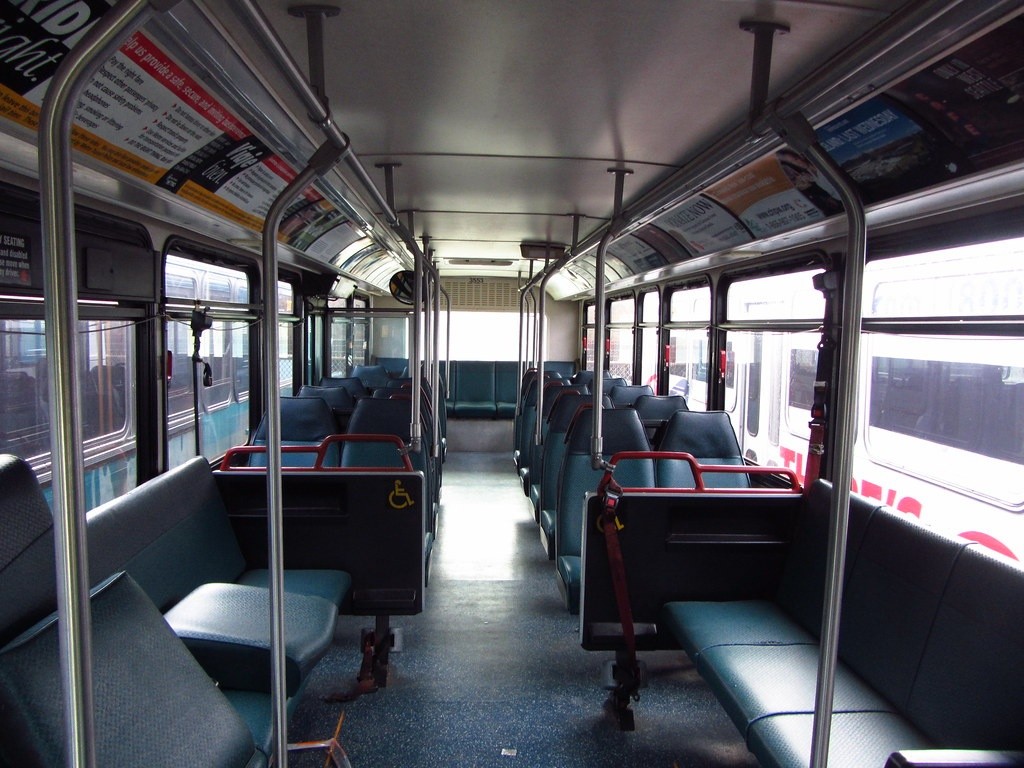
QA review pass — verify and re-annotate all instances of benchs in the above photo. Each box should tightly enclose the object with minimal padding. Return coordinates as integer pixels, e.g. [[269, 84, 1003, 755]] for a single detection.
[[0, 356, 1024, 767]]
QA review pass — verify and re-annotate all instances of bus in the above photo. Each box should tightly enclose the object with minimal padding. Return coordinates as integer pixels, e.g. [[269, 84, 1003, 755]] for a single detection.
[[585, 234, 1024, 577], [0, 253, 368, 530]]
